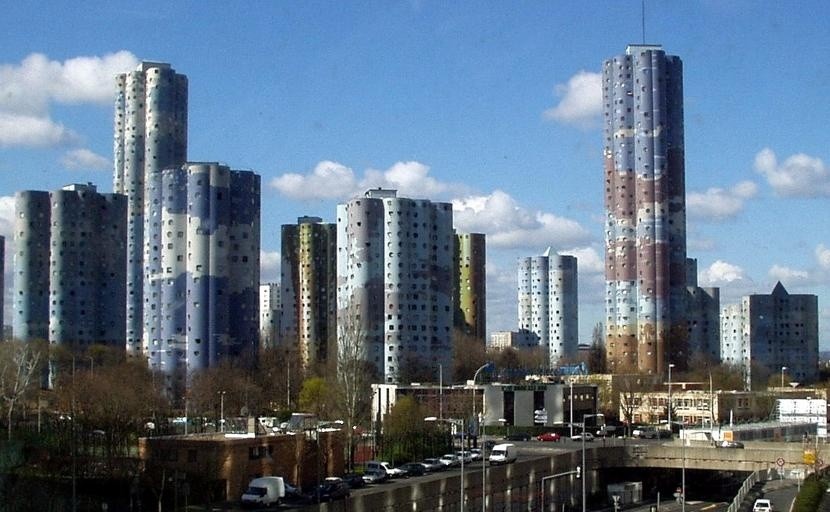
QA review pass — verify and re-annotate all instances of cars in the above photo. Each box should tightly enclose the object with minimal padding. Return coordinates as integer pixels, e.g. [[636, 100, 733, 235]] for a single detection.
[[504, 432, 532, 443], [653, 430, 672, 439], [166, 411, 346, 435], [538, 432, 560, 443], [239, 436, 503, 507], [716, 441, 745, 449], [752, 499, 774, 512], [571, 433, 595, 441]]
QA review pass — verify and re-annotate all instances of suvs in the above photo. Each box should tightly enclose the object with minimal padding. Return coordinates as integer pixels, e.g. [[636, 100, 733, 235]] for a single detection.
[[634, 426, 654, 439]]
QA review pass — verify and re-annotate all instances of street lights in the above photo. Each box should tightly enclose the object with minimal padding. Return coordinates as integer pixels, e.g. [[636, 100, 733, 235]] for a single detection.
[[540, 466, 581, 512], [582, 414, 604, 512], [472, 362, 496, 420], [180, 395, 188, 433], [481, 418, 506, 512], [781, 365, 789, 397], [667, 363, 675, 429], [437, 362, 444, 418], [569, 367, 580, 440], [216, 389, 227, 432], [424, 413, 464, 512]]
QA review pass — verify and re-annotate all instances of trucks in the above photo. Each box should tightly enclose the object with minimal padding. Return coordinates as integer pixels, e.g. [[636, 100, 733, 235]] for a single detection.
[[488, 443, 517, 465]]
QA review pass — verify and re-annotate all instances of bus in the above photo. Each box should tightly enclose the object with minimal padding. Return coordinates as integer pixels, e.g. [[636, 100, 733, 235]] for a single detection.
[[607, 481, 643, 505]]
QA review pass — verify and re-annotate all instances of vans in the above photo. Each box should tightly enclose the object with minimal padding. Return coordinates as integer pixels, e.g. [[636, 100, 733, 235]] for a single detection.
[[789, 470, 800, 478]]
